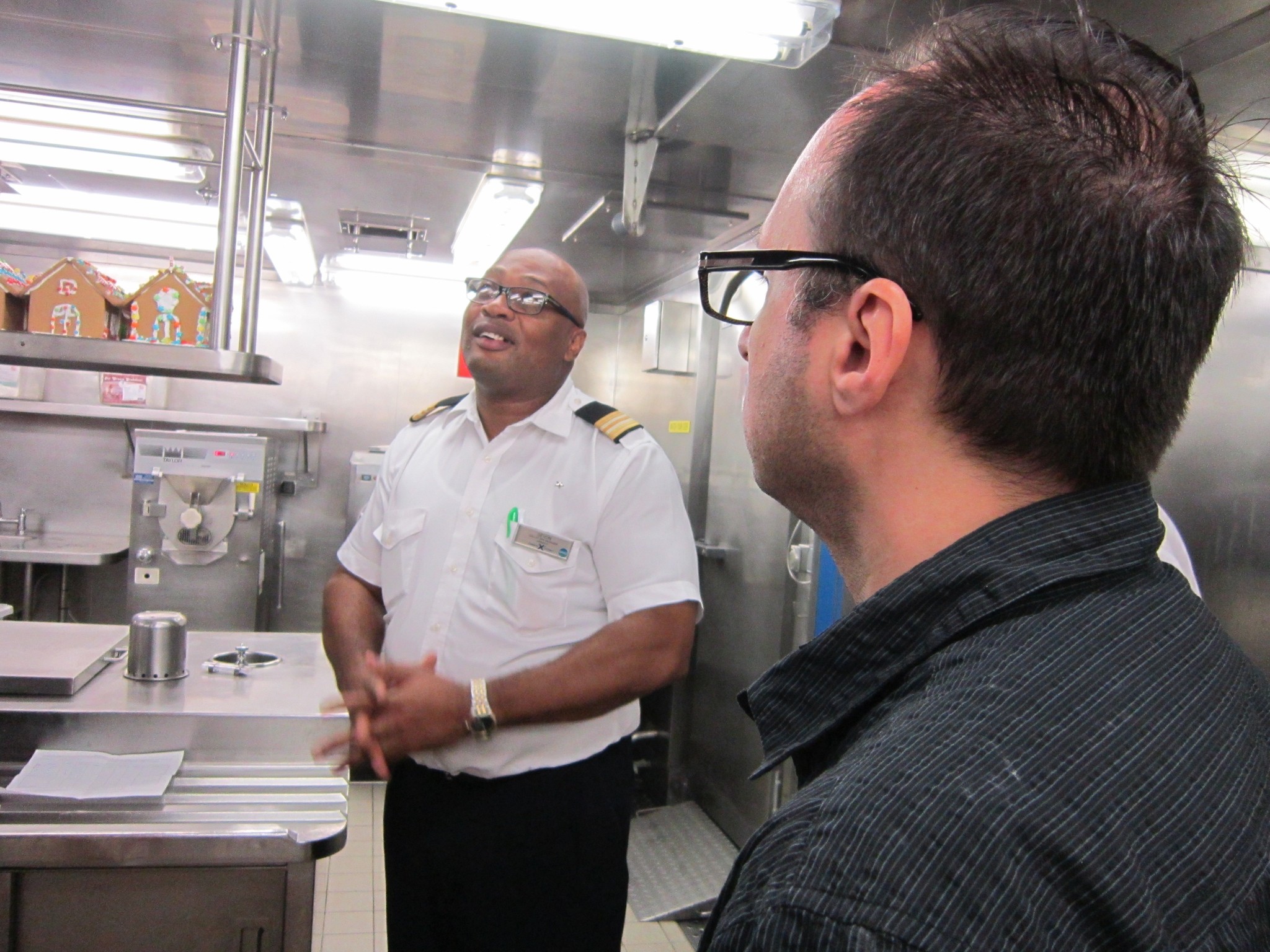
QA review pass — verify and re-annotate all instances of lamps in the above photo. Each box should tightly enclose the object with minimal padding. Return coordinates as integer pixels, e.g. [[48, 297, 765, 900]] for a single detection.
[[0, 119, 214, 184], [448, 169, 547, 272], [263, 197, 318, 286], [379, 0, 844, 69]]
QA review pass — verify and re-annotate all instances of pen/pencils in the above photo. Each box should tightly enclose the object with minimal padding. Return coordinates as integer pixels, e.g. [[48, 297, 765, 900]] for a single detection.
[[506, 507, 519, 538]]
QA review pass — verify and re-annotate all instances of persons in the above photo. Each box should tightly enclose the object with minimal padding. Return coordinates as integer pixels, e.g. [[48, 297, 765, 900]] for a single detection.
[[648, 2, 1270, 952], [321, 242, 707, 952]]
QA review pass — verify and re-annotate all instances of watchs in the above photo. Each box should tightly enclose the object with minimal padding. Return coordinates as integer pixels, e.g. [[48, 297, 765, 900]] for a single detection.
[[464, 677, 499, 743]]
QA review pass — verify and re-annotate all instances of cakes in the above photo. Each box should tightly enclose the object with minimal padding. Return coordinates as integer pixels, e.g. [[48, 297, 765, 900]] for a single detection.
[[0, 257, 213, 347]]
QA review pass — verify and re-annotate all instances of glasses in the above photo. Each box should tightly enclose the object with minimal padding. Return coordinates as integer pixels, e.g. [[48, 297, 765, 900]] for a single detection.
[[699, 251, 922, 324], [466, 277, 584, 329]]
